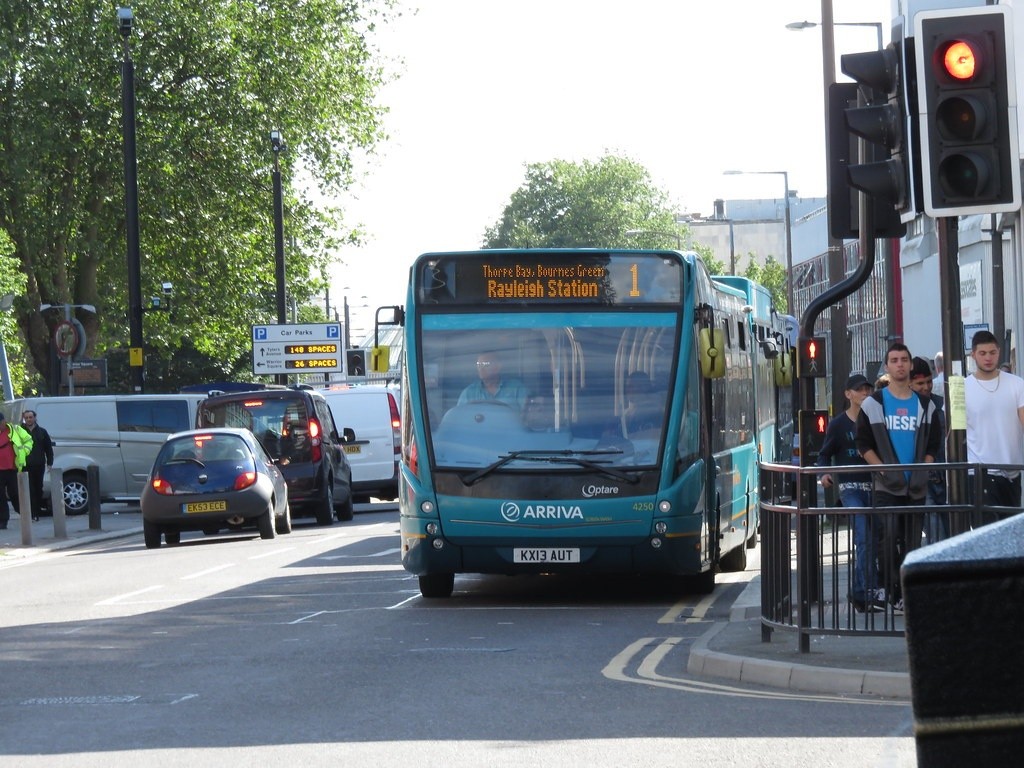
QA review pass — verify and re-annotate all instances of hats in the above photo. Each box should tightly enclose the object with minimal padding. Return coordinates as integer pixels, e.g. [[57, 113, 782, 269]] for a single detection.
[[844, 375, 874, 390], [909, 355, 932, 379]]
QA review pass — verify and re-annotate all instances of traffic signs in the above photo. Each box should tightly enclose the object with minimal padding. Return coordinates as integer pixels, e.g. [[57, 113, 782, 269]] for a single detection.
[[250, 319, 343, 375]]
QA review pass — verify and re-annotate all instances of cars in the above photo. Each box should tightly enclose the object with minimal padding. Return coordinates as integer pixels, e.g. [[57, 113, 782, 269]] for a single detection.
[[138, 425, 292, 548]]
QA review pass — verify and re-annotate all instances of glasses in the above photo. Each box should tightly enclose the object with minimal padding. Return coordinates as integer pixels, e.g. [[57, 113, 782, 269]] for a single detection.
[[475, 360, 493, 369]]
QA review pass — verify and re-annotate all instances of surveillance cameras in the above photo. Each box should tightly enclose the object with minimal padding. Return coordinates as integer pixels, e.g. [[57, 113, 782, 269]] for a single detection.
[[118, 8, 133, 27], [163, 282, 173, 296]]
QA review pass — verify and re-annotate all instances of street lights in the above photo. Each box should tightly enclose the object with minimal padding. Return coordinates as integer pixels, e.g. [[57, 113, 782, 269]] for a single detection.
[[784, 18, 897, 352], [625, 228, 684, 251], [113, 6, 176, 395], [675, 216, 735, 276], [342, 285, 352, 349], [723, 168, 796, 318], [39, 303, 98, 396], [268, 129, 289, 386]]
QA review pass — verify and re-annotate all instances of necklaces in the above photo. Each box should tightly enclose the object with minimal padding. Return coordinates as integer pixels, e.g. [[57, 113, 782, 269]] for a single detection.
[[976, 371, 999, 393]]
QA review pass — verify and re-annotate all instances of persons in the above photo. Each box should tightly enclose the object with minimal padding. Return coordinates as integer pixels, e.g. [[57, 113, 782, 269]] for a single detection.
[[19, 411, 53, 521], [0, 413, 34, 529], [456, 350, 532, 411], [611, 371, 664, 434], [818, 344, 945, 614], [964, 331, 1024, 524]]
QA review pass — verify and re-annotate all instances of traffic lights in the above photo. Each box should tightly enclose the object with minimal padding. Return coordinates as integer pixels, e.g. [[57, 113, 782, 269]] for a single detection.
[[913, 3, 1024, 220], [797, 409, 830, 458], [839, 15, 917, 223], [794, 336, 827, 380]]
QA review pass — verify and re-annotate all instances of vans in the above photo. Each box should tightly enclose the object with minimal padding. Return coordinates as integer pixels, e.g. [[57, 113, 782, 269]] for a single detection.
[[303, 377, 403, 503], [0, 392, 210, 513]]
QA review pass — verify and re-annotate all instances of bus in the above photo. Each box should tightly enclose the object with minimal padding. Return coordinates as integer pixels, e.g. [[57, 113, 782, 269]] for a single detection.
[[708, 274, 801, 534], [369, 245, 758, 597]]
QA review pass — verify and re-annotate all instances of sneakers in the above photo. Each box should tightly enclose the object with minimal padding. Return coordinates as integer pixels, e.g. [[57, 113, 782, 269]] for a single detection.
[[867, 587, 889, 612], [888, 595, 905, 616]]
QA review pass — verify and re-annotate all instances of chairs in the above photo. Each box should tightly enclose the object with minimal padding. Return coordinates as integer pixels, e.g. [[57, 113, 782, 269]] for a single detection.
[[174, 450, 197, 462], [224, 448, 245, 459]]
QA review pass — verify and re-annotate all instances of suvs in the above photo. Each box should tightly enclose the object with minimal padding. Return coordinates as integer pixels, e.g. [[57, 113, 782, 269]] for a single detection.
[[194, 382, 355, 526]]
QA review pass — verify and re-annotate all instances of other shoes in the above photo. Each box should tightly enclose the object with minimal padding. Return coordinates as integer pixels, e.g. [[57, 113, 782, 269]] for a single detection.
[[846, 591, 865, 613]]
